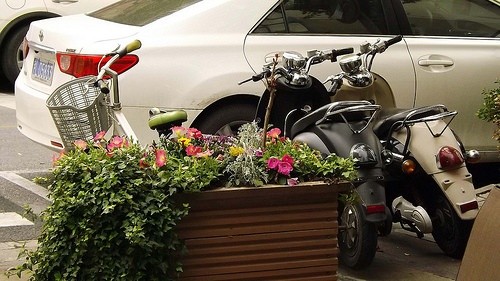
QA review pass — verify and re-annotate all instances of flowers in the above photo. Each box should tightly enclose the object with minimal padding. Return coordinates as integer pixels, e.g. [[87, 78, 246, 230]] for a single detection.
[[3, 120, 360, 281]]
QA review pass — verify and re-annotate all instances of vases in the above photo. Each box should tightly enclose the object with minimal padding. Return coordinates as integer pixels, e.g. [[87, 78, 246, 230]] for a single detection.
[[171, 179, 352, 281]]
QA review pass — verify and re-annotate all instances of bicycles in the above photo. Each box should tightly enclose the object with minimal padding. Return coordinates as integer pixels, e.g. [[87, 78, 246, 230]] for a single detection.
[[45, 39, 189, 154]]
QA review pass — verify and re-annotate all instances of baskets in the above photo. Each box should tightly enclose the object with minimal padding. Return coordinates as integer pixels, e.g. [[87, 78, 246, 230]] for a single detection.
[[45, 75, 108, 149]]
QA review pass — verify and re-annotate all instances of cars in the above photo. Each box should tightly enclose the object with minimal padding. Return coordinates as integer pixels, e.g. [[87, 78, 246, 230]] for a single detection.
[[0, 0, 121, 93], [12, 0, 500, 167]]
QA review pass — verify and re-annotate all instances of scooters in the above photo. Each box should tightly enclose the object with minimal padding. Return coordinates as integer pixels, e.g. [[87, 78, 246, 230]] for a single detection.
[[238, 47, 393, 270], [323, 33, 480, 258]]
